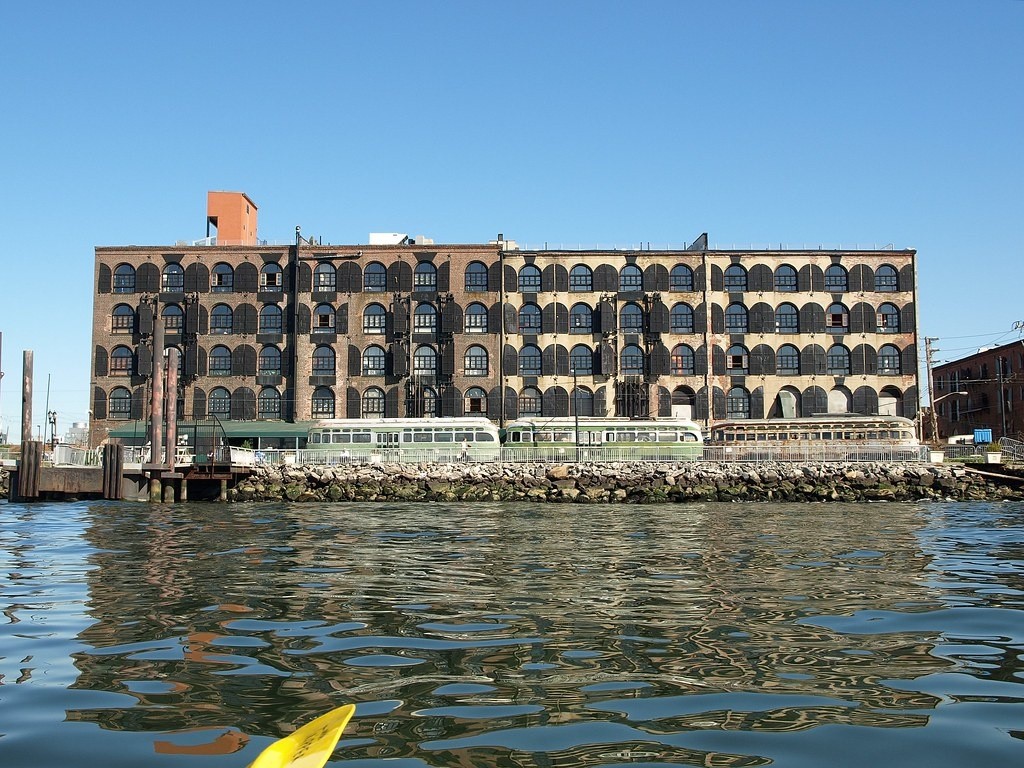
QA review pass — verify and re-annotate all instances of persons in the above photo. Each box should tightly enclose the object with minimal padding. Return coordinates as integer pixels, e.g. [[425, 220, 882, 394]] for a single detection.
[[340, 447, 349, 464], [461, 437, 468, 462], [206, 450, 213, 464]]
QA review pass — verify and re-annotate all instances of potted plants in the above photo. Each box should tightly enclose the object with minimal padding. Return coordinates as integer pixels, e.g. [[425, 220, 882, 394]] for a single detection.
[[927, 440, 945, 464], [982, 444, 1002, 464]]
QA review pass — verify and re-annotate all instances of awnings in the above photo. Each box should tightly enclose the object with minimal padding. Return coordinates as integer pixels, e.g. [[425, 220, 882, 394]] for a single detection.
[[108, 419, 314, 438]]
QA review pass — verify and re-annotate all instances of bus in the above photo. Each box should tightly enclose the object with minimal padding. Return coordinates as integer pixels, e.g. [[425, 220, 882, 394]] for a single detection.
[[306, 415, 502, 468], [500, 414, 705, 465], [705, 411, 920, 461]]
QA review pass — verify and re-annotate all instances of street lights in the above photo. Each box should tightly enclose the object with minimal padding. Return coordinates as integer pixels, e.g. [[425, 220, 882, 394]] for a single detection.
[[37, 425, 40, 442], [929, 391, 969, 444], [570, 360, 583, 465], [48, 410, 57, 451]]
